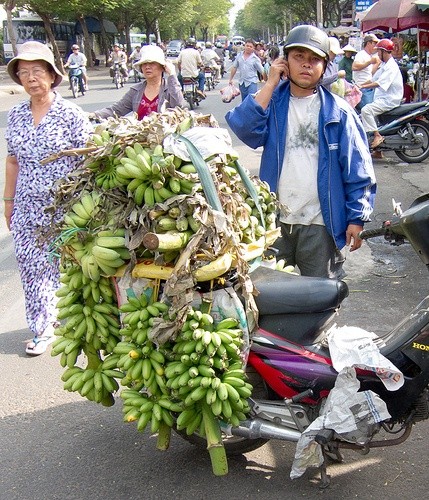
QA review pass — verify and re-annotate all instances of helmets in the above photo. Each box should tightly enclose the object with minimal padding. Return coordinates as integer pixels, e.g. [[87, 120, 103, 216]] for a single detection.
[[373, 39, 396, 63], [71, 44, 79, 49]]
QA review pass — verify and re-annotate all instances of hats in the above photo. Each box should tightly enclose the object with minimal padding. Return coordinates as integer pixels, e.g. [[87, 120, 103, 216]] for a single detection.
[[363, 34, 379, 43], [132, 46, 174, 75], [341, 45, 358, 54], [282, 26, 331, 90], [328, 36, 346, 56], [6, 41, 64, 90]]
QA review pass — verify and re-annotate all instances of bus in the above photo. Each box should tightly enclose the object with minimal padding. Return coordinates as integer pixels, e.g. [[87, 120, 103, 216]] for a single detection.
[[2, 19, 75, 62], [115, 33, 156, 48], [215, 35, 228, 48]]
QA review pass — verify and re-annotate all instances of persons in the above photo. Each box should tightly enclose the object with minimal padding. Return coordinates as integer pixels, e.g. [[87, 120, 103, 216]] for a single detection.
[[0, 40, 91, 355], [224, 23, 378, 276], [127, 45, 142, 65], [318, 36, 346, 94], [337, 44, 357, 83], [63, 43, 88, 90], [350, 33, 380, 110], [106, 42, 130, 83], [176, 35, 285, 104], [358, 39, 405, 149], [87, 43, 191, 122]]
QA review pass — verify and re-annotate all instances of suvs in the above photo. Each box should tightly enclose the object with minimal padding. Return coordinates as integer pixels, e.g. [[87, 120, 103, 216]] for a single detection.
[[166, 39, 185, 57]]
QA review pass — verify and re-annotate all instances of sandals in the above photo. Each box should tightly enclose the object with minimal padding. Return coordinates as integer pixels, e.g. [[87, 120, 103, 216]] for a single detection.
[[25, 334, 58, 355]]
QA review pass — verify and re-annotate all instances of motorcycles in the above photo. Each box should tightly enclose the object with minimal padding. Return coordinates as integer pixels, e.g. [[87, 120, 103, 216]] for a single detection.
[[107, 58, 127, 89], [128, 57, 142, 83], [342, 85, 429, 164], [217, 55, 227, 77], [229, 46, 237, 61], [204, 59, 221, 91], [63, 63, 87, 99], [123, 192, 429, 462], [179, 64, 207, 110]]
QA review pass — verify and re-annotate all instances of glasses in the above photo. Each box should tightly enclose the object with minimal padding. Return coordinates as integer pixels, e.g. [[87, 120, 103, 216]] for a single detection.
[[16, 68, 49, 79]]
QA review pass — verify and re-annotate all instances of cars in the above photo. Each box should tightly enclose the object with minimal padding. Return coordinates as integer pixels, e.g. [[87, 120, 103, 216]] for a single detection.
[[205, 42, 213, 49]]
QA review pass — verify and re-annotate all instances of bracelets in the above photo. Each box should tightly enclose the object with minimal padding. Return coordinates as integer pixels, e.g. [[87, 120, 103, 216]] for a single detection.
[[2, 198, 15, 201]]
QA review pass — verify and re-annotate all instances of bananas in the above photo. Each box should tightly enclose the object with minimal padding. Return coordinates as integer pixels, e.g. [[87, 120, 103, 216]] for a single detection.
[[51, 113, 280, 443]]
